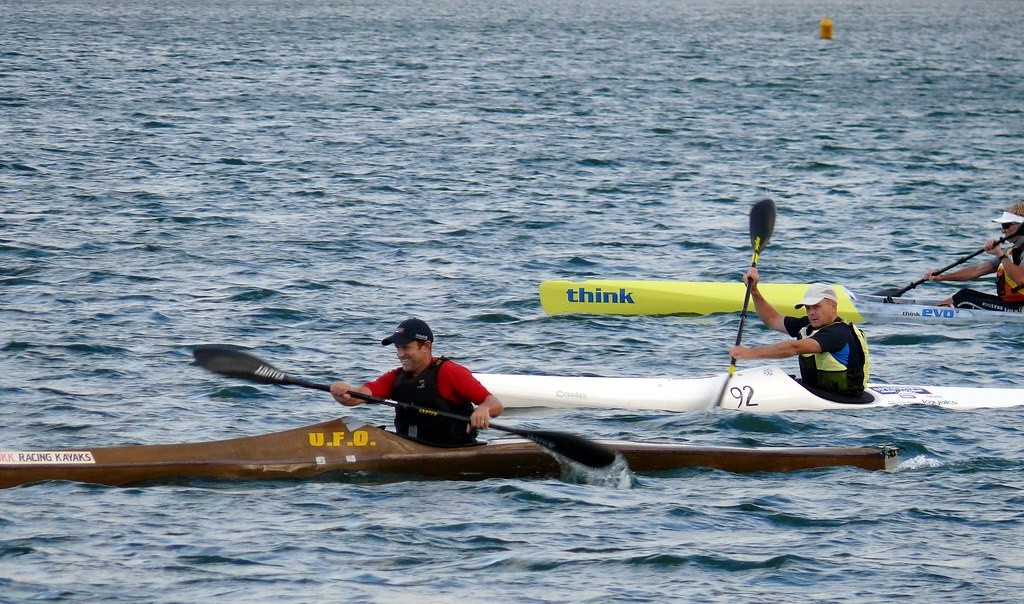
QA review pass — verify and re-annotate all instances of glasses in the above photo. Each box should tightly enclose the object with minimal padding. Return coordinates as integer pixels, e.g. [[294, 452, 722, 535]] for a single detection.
[[1002, 223, 1020, 229]]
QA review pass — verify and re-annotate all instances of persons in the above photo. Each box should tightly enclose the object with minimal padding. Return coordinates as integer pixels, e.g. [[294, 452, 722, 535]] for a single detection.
[[330, 318, 504, 447], [924, 203, 1024, 312], [730, 267, 870, 404]]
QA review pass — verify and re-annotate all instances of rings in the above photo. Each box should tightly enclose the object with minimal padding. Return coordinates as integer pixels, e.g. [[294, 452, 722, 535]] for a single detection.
[[481, 420, 484, 423]]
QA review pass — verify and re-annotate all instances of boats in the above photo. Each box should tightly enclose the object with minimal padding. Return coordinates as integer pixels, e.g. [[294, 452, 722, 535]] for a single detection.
[[538, 280, 1024, 328], [1, 414, 898, 489], [466, 366, 1024, 416]]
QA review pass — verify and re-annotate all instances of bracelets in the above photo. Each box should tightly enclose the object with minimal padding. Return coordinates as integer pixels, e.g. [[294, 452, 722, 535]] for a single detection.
[[999, 254, 1008, 260]]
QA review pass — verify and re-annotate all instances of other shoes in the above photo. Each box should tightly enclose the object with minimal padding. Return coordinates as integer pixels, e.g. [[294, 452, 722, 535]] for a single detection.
[[883, 296, 894, 303]]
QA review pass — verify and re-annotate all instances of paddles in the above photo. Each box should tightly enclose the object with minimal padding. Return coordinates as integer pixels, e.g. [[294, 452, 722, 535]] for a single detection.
[[872, 220, 1024, 298], [711, 197, 778, 410], [193, 342, 617, 469]]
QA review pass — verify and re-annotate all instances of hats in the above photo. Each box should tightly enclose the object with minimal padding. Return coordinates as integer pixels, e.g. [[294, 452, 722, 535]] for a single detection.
[[793, 284, 838, 309], [382, 317, 433, 346], [992, 211, 1024, 224]]
